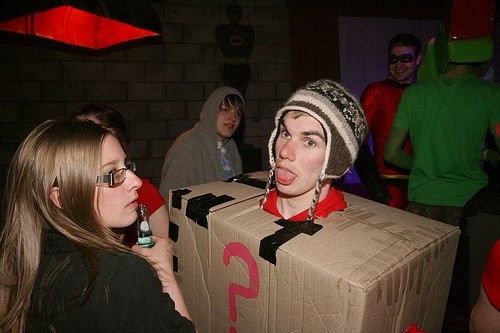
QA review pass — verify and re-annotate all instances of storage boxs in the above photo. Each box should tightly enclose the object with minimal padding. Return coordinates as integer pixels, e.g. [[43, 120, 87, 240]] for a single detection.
[[169, 171, 462, 333]]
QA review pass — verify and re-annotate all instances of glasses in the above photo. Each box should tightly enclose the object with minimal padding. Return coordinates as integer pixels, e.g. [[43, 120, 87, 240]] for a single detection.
[[52, 161, 136, 187]]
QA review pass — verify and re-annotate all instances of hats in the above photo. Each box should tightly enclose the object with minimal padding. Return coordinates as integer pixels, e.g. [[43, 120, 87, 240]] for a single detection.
[[261, 79, 368, 220], [448, 0, 494, 63]]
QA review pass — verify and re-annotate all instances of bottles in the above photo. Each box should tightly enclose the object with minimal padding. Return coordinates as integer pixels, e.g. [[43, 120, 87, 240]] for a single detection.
[[136, 204, 154, 248]]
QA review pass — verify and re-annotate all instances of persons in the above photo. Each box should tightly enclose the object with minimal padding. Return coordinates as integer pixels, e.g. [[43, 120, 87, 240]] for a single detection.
[[0, 116, 197, 333], [469, 239, 500, 333], [360, 33, 423, 210], [73, 104, 169, 239], [383, 33, 500, 227], [257, 81, 369, 221], [158, 86, 246, 211], [216, 4, 255, 150]]
